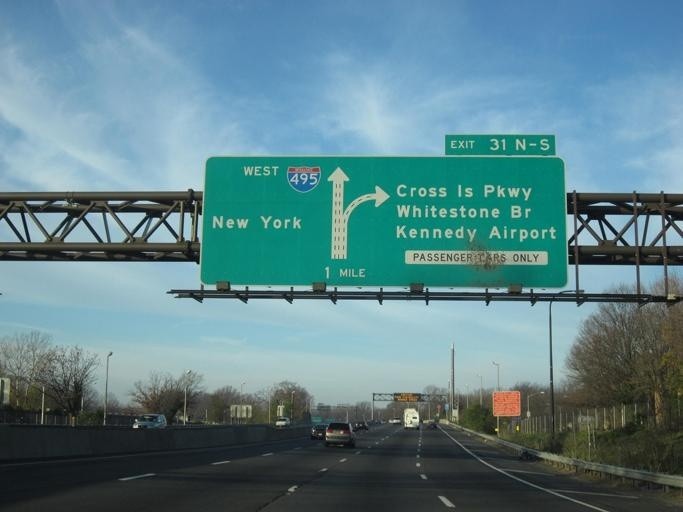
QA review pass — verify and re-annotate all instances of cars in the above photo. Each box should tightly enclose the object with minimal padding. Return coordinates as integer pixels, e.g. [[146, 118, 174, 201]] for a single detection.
[[375, 415, 401, 425], [311, 420, 369, 448]]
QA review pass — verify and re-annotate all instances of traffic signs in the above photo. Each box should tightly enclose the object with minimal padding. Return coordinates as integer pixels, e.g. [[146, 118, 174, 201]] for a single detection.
[[200, 154, 570, 292], [445, 132, 558, 158]]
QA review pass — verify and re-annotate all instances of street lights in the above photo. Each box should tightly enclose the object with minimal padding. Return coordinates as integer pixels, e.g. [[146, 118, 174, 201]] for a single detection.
[[290, 390, 296, 426], [182, 383, 193, 427], [527, 390, 546, 420], [104, 351, 113, 425], [456, 361, 501, 440], [240, 381, 246, 425], [549, 290, 585, 444]]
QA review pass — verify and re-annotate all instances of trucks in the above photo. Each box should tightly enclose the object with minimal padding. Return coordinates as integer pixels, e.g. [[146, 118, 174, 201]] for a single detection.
[[403, 408, 420, 430]]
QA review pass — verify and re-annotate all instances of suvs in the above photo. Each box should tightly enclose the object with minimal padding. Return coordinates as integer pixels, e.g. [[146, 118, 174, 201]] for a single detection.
[[276, 417, 291, 426], [131, 413, 168, 429]]
